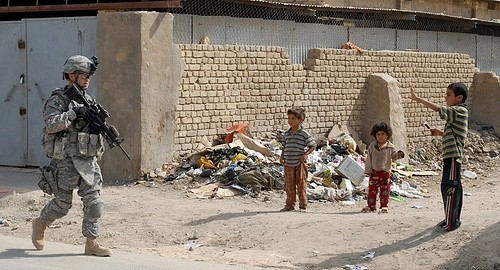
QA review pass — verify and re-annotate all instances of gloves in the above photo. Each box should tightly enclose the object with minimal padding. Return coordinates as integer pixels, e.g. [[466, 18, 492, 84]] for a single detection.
[[73, 106, 86, 117]]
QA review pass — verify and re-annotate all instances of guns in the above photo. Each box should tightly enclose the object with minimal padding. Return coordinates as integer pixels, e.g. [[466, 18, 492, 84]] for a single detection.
[[63, 83, 133, 161]]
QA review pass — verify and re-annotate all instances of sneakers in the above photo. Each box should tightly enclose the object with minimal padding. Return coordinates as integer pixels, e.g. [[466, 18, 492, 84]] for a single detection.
[[85, 237, 112, 257], [32, 218, 45, 250]]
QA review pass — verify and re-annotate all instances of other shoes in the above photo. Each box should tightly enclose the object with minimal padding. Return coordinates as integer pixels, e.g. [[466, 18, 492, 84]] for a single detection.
[[361, 207, 376, 212], [280, 208, 294, 212], [381, 207, 388, 212], [299, 208, 306, 213]]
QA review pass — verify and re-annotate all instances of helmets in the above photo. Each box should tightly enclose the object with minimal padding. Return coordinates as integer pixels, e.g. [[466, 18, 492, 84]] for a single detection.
[[62, 55, 95, 75]]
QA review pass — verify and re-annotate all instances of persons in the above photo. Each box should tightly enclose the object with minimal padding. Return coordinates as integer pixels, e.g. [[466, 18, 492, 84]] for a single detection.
[[280, 107, 316, 212], [32, 55, 119, 257], [408, 82, 469, 232], [361, 122, 405, 213]]
